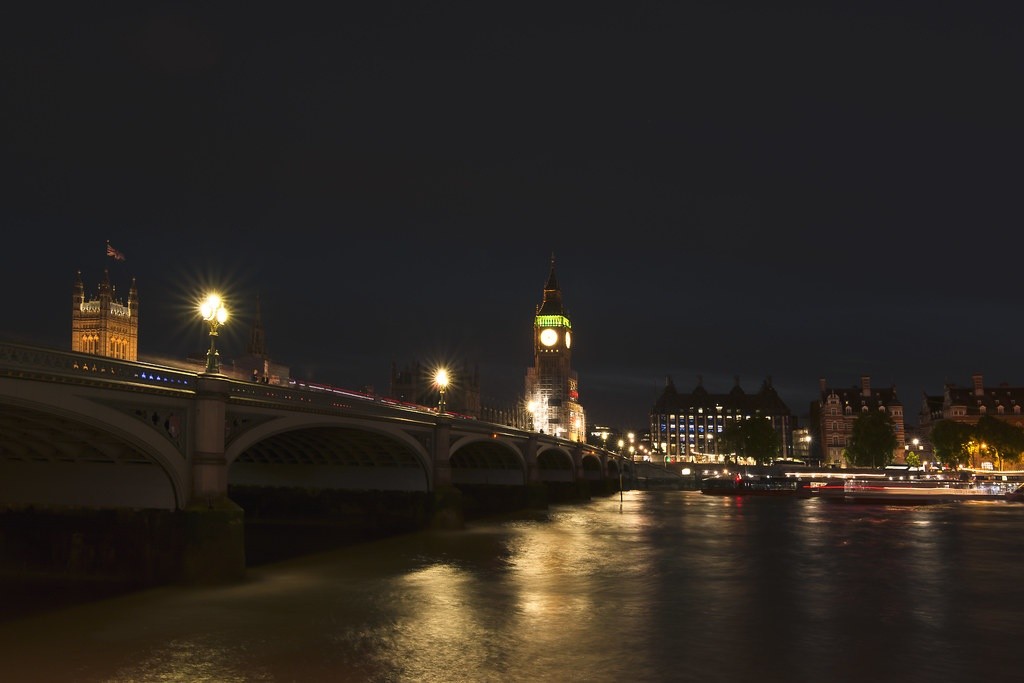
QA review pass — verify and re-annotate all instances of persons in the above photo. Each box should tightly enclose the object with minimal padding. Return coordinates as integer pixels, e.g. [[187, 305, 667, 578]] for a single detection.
[[250, 369, 259, 384], [261, 374, 269, 385]]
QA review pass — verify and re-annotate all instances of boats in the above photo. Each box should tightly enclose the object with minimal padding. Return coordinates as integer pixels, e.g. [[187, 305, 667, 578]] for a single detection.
[[701, 469, 798, 491], [843, 464, 1024, 500]]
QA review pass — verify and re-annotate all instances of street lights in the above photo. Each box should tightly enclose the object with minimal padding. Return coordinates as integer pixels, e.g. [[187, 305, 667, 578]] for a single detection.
[[200, 293, 228, 373], [913, 439, 918, 451], [435, 369, 448, 417]]
